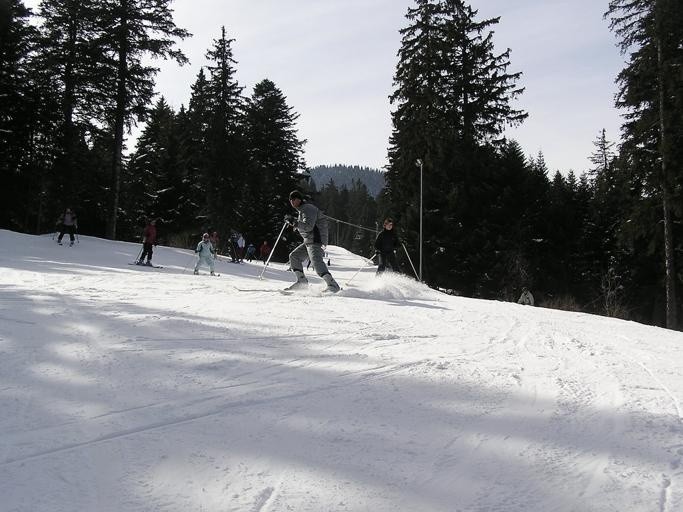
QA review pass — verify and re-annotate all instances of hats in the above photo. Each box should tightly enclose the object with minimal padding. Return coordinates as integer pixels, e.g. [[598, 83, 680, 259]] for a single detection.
[[289, 190, 303, 201], [203, 233, 209, 239]]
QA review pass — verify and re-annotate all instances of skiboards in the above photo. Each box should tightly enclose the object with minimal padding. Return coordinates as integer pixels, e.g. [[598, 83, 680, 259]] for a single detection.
[[194, 272, 220, 277], [128, 261, 163, 269], [233, 285, 338, 296], [56, 238, 76, 248]]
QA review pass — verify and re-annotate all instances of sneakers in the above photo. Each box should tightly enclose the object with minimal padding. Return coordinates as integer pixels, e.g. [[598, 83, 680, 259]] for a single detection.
[[210, 271, 215, 276], [146, 260, 152, 266], [139, 259, 144, 266], [194, 268, 198, 275]]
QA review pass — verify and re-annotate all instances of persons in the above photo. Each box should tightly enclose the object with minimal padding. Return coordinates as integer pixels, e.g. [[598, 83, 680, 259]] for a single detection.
[[136, 216, 156, 265], [374, 218, 403, 279], [518, 286, 535, 306], [193, 229, 274, 276], [56, 205, 79, 247], [285, 191, 340, 295]]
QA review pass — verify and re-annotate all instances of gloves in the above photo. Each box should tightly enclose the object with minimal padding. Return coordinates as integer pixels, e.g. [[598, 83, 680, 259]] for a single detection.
[[285, 215, 293, 226], [375, 249, 382, 257]]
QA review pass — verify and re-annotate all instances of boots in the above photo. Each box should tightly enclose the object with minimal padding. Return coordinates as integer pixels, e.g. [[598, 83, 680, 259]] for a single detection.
[[320, 272, 340, 294], [284, 269, 308, 290]]
